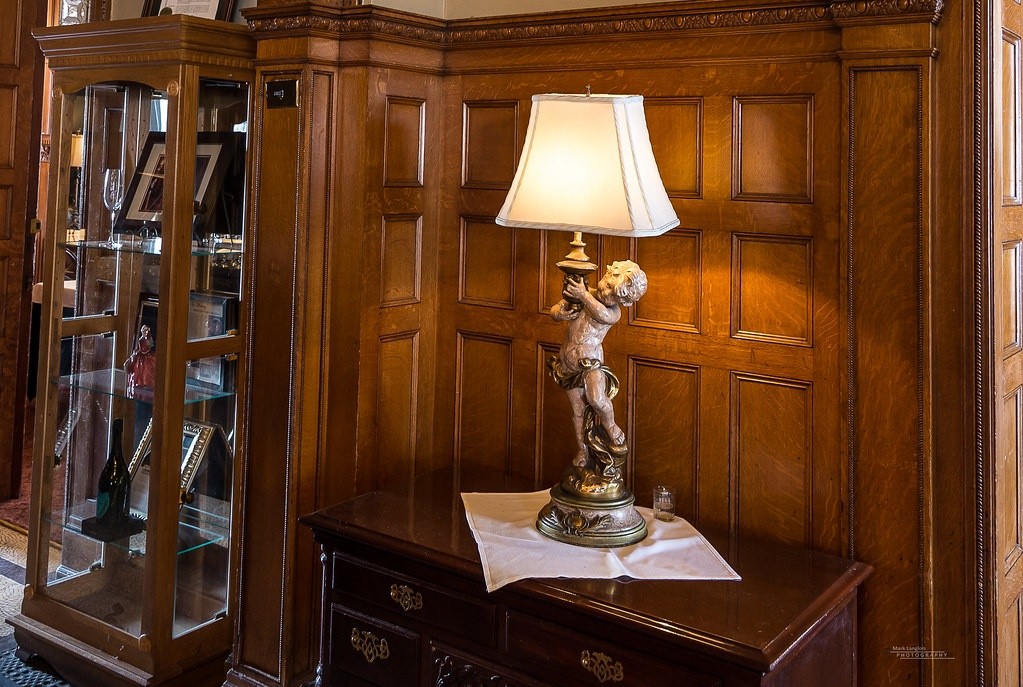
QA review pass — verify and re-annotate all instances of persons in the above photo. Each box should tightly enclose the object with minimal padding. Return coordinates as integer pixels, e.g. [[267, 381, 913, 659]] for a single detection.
[[550, 260, 647, 467], [128, 325, 155, 388]]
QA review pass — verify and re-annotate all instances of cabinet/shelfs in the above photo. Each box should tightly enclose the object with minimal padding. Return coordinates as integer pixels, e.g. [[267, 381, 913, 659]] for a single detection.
[[8, 11, 257, 687], [297, 458, 875, 687]]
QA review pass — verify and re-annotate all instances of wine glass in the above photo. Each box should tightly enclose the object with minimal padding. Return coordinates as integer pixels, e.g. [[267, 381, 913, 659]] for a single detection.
[[98, 168, 126, 249]]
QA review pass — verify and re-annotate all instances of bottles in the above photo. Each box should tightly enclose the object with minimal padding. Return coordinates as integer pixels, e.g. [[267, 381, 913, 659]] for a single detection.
[[95, 417, 132, 537]]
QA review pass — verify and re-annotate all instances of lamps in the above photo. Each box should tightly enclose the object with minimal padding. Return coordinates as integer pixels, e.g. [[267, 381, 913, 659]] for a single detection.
[[496, 82, 684, 549]]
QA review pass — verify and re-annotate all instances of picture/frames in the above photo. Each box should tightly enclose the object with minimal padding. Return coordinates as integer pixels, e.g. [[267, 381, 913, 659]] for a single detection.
[[112, 130, 236, 242], [126, 416, 217, 525], [130, 290, 160, 359], [201, 130, 247, 237], [186, 292, 235, 392], [185, 417, 232, 486]]
